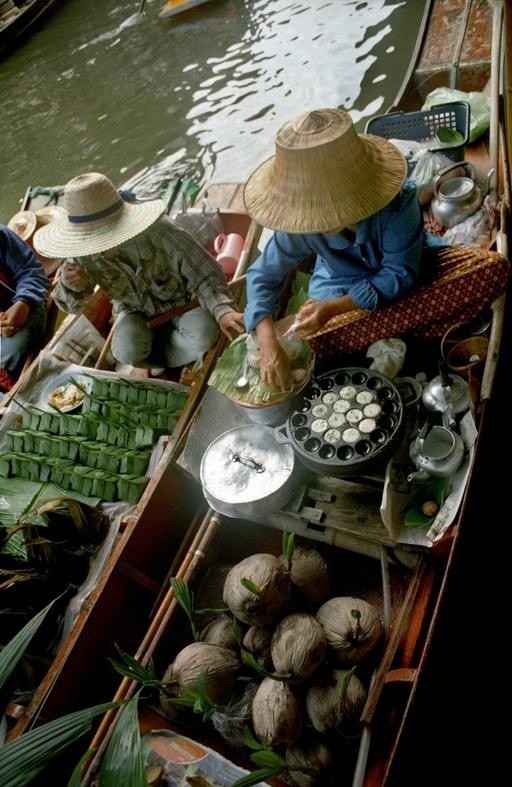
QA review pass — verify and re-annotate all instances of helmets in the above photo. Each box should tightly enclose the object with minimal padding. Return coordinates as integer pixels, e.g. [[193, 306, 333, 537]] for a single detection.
[[30, 169, 170, 260]]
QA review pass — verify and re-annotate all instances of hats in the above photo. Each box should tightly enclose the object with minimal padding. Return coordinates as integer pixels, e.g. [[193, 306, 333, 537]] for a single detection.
[[242, 107, 411, 235], [4, 208, 39, 244]]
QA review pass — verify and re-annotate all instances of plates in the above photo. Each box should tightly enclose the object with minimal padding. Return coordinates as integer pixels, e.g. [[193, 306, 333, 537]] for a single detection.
[[40, 371, 94, 415]]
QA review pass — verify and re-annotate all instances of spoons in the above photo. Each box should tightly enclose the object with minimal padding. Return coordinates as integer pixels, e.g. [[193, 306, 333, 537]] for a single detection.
[[233, 351, 249, 389]]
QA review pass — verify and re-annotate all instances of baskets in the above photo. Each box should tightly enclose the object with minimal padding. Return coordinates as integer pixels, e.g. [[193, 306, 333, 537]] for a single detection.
[[363, 100, 472, 180]]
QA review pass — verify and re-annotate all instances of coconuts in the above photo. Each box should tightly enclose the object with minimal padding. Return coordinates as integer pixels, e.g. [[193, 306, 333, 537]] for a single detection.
[[160, 546, 383, 787]]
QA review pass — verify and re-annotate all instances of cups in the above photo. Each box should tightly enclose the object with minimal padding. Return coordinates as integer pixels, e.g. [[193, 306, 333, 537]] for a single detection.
[[213, 233, 244, 274]]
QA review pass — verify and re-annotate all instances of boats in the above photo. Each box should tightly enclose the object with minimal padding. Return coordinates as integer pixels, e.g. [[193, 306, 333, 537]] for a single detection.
[[156, 0, 211, 17], [376, 1, 512, 257], [0, 0, 58, 62]]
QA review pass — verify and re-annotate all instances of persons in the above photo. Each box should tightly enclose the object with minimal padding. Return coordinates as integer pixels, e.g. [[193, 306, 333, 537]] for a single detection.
[[241, 184, 448, 392], [50, 217, 251, 375], [0, 223, 59, 385]]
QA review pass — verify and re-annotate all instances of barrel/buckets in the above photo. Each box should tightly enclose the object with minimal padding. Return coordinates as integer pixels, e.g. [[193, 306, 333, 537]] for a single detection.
[[228, 332, 316, 427]]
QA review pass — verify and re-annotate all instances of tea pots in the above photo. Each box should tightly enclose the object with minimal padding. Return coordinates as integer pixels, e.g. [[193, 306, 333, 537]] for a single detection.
[[407, 409, 466, 483], [429, 160, 496, 228], [423, 360, 472, 426]]
